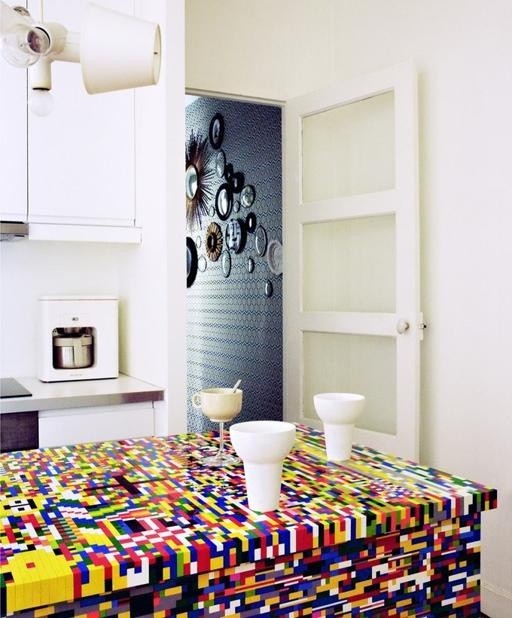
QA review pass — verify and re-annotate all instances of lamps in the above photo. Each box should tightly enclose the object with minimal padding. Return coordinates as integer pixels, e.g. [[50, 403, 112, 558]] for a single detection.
[[0, 0, 162, 95]]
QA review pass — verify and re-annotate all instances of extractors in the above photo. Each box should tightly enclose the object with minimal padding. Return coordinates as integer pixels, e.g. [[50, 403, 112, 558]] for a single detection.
[[0, 222, 30, 242]]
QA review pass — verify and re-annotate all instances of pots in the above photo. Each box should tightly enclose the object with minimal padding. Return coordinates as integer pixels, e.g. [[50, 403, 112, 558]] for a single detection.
[[53, 334, 94, 369]]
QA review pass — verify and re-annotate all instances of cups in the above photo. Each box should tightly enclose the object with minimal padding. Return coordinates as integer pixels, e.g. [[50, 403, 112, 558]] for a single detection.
[[313, 393, 366, 462], [229, 420, 296, 511]]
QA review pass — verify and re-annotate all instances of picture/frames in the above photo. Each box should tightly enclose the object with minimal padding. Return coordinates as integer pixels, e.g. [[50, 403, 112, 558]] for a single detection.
[[266, 241, 283, 275], [209, 113, 224, 151]]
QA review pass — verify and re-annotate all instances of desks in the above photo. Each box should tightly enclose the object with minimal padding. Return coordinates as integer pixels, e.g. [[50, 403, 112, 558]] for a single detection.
[[0, 422, 497, 618]]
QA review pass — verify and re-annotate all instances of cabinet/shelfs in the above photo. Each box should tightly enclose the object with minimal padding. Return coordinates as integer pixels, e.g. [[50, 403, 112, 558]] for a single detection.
[[0, 0, 142, 245]]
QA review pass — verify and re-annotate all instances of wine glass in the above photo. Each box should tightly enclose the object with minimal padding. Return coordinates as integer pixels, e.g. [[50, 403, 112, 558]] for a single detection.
[[191, 387, 244, 466]]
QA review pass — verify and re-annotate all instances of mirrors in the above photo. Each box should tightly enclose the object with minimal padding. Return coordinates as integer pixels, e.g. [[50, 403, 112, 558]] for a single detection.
[[187, 237, 196, 289], [185, 129, 215, 231], [216, 184, 232, 221]]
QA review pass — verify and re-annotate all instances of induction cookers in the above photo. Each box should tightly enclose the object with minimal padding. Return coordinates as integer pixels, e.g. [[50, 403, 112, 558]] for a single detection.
[[1, 377, 33, 399]]
